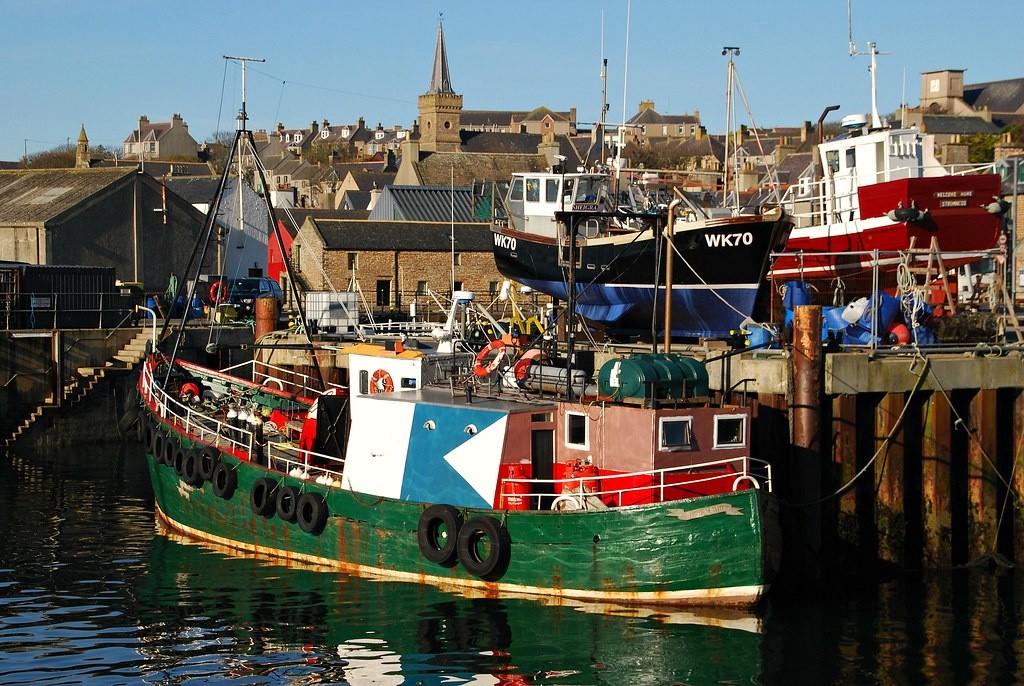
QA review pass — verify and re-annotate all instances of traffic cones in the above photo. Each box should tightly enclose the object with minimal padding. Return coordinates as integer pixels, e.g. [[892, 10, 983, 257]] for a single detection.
[[774, 292, 791, 338]]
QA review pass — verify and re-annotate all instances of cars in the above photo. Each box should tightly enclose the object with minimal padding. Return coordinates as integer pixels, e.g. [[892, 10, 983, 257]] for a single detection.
[[223, 276, 282, 314]]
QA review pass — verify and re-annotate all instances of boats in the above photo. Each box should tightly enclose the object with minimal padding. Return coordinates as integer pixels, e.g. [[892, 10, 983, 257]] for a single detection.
[[490, 0, 795, 340], [134, 55, 771, 605], [765, 0, 1001, 290]]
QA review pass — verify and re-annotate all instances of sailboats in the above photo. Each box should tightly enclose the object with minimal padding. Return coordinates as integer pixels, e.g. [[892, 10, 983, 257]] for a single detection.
[[122, 515, 779, 686]]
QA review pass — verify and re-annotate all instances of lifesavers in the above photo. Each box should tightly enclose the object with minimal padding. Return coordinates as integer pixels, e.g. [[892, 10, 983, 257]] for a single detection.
[[211, 282, 228, 301], [418, 504, 460, 563], [276, 487, 301, 524], [298, 495, 327, 535], [135, 412, 236, 498], [370, 371, 395, 396], [455, 519, 505, 579], [472, 341, 506, 376], [250, 477, 278, 515]]
